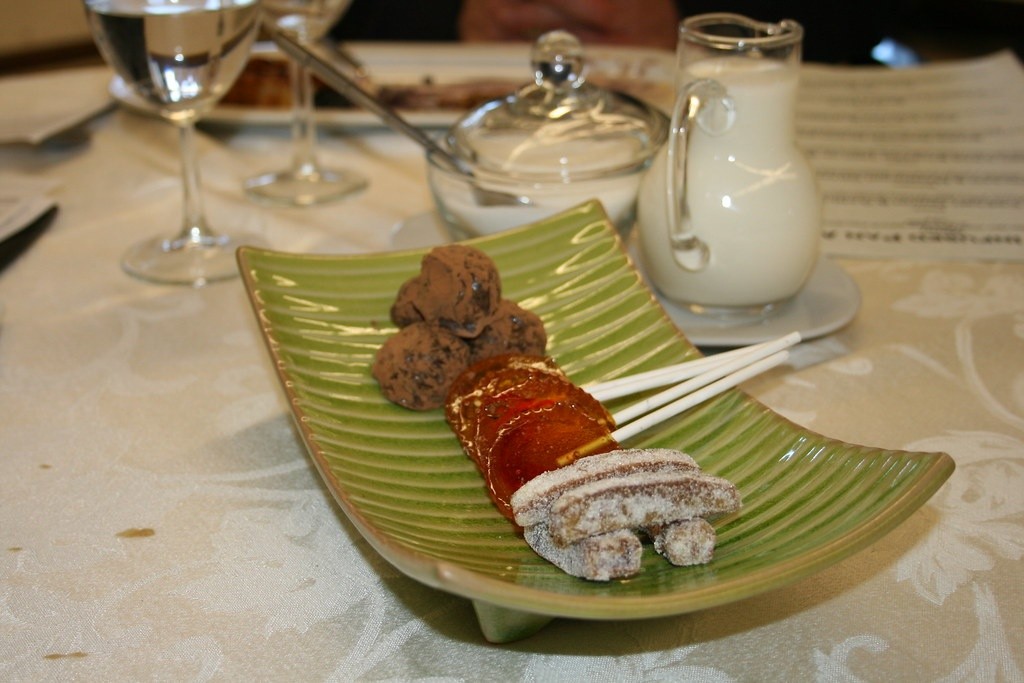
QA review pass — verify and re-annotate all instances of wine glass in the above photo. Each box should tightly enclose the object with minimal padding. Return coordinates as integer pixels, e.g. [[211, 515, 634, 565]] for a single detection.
[[250, 0, 368, 204], [83, 1, 261, 283]]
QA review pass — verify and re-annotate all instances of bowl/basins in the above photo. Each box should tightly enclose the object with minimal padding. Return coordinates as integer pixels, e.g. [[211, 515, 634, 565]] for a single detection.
[[423, 30, 668, 244]]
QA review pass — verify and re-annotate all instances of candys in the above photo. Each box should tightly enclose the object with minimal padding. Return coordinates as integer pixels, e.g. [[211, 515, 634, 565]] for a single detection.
[[439, 332, 804, 524]]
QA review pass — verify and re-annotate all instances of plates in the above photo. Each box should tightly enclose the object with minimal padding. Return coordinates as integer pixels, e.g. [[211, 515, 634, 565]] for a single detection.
[[234, 198, 955, 643], [111, 58, 679, 126], [626, 243, 862, 346]]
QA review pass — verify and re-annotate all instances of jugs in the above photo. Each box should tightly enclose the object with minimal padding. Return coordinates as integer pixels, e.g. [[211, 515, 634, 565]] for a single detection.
[[637, 12, 820, 314]]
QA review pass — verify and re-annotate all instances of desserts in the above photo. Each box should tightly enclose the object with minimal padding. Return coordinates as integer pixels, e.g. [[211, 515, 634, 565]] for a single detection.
[[373, 243, 547, 409]]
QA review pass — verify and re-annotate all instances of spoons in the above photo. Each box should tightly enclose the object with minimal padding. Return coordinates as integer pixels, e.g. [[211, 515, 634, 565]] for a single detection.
[[271, 30, 535, 210]]
